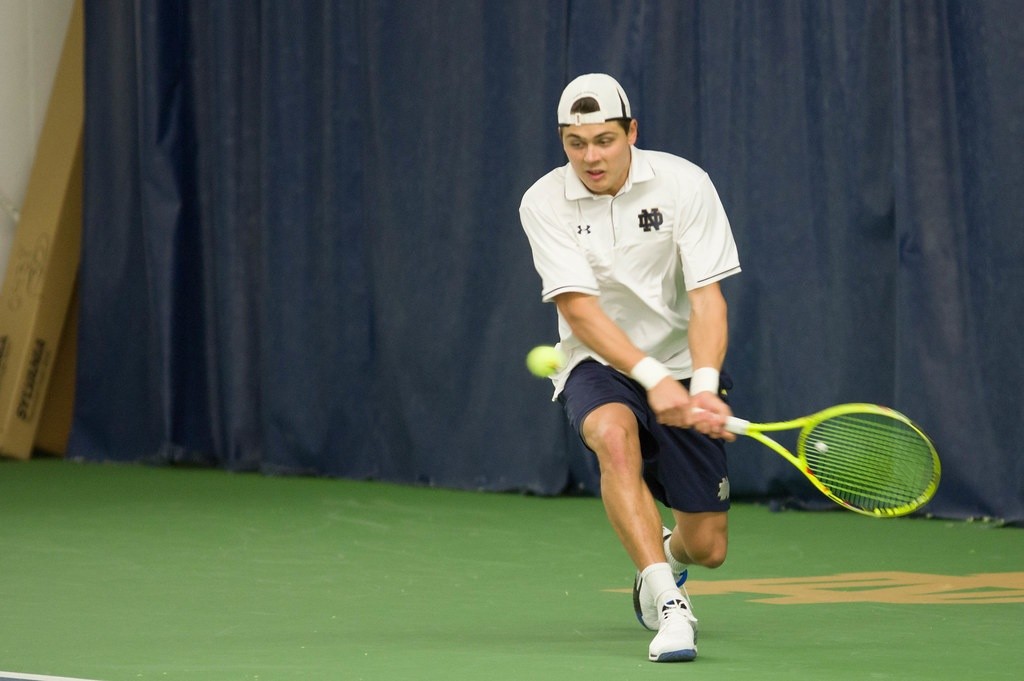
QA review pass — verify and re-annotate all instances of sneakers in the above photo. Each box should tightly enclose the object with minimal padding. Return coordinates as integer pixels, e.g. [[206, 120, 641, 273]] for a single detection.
[[632, 524, 696, 632], [648, 593, 699, 662]]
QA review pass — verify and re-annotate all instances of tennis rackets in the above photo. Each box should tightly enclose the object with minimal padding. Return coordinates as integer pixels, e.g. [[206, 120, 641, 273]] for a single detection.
[[687, 401, 943, 518]]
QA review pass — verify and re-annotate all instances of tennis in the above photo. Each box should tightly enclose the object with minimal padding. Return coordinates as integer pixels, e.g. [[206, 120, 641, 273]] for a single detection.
[[526, 344, 565, 377]]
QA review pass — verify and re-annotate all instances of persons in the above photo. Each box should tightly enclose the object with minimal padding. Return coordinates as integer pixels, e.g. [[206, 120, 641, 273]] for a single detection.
[[518, 72, 742, 663]]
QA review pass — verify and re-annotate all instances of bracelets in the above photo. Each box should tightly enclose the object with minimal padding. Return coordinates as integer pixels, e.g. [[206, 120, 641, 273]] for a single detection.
[[688, 367, 721, 396], [630, 357, 673, 391]]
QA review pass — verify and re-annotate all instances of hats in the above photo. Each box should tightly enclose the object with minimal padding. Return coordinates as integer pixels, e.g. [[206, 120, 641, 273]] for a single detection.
[[557, 72, 632, 124]]
